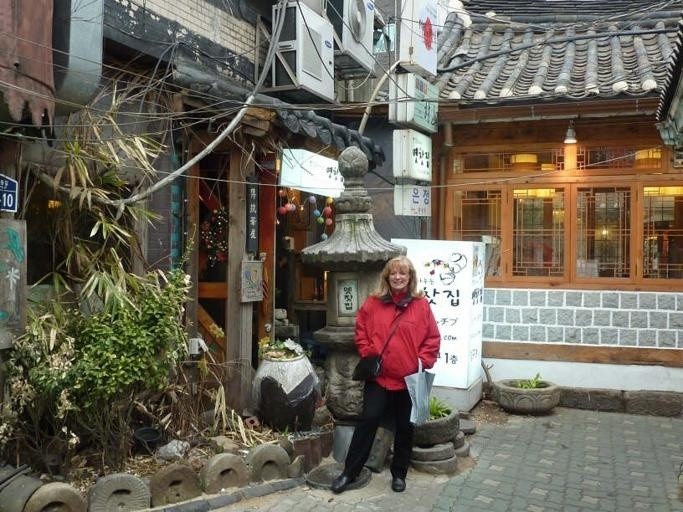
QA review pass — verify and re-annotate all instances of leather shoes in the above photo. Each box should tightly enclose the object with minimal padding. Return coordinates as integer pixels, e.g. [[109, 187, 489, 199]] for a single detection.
[[392, 478, 406, 492], [332, 476, 356, 492]]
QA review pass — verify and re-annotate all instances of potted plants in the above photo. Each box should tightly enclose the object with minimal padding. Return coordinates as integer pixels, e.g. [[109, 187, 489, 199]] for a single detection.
[[494, 379, 561, 415], [410, 396, 460, 445]]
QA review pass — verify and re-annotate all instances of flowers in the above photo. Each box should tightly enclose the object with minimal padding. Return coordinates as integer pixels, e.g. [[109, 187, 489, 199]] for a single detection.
[[255, 333, 312, 358]]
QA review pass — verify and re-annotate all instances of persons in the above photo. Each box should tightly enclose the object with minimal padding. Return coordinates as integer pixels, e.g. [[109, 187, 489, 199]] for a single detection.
[[331, 254, 442, 495], [526, 225, 553, 275]]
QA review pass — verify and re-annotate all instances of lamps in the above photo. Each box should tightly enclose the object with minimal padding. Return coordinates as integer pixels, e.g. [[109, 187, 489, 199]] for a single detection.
[[562, 119, 578, 144], [508, 152, 556, 200], [633, 148, 682, 198]]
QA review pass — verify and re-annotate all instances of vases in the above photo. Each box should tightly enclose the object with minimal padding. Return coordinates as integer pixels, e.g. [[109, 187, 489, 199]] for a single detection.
[[250, 359, 319, 439]]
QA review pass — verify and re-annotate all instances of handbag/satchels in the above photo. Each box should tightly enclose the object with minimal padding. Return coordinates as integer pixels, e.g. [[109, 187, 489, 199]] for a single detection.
[[405, 358, 436, 427], [352, 354, 381, 381]]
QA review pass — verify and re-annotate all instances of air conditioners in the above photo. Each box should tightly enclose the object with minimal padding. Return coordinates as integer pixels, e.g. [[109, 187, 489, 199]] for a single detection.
[[269, 2, 334, 102], [325, 0, 375, 74]]
